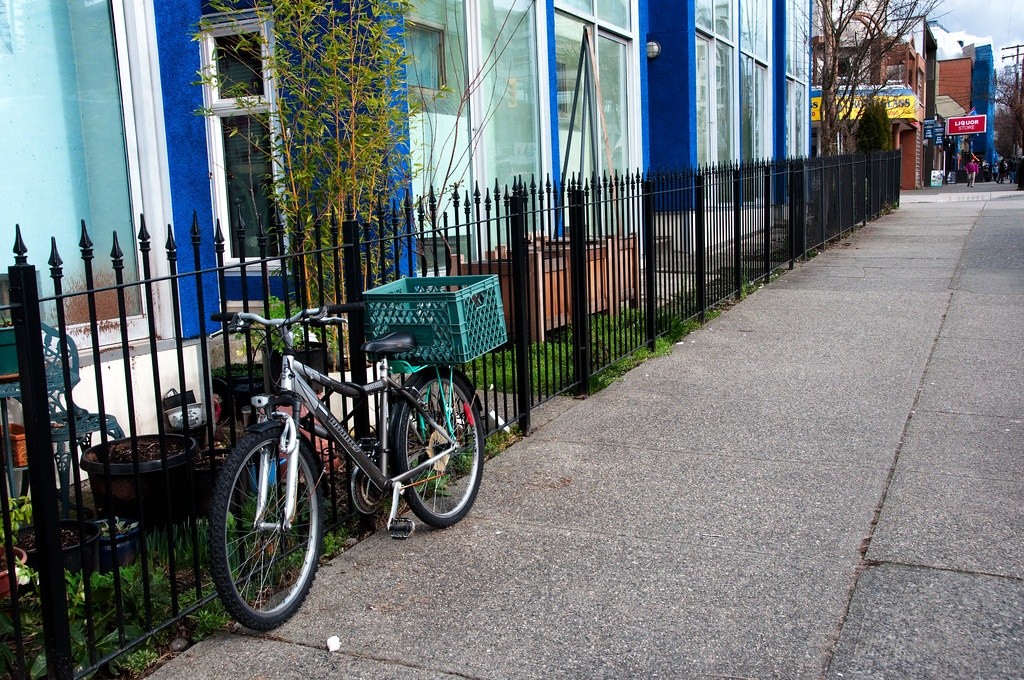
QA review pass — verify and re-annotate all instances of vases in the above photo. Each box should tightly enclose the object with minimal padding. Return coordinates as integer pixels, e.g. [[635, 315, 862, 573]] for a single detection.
[[0, 390, 286, 588]]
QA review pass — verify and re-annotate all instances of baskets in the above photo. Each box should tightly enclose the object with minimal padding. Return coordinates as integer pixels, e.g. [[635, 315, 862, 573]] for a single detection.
[[361, 274, 508, 363]]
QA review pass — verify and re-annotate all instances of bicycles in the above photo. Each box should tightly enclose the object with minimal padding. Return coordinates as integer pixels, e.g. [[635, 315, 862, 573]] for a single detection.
[[207, 302, 488, 630]]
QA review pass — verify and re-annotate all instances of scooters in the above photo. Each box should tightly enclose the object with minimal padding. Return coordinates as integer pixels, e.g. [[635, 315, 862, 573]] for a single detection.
[[983, 164, 1012, 184]]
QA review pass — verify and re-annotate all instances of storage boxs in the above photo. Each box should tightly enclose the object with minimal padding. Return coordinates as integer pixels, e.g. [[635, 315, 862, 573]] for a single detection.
[[363, 274, 508, 363]]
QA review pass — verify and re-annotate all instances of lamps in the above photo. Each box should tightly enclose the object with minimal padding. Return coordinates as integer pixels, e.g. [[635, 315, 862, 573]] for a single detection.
[[647, 41, 661, 59]]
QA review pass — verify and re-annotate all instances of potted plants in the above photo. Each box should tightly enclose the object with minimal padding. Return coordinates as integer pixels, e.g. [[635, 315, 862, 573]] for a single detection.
[[93, 519, 140, 577], [0, 491, 32, 603], [212, 363, 265, 420], [236, 295, 328, 395], [0, 315, 19, 374]]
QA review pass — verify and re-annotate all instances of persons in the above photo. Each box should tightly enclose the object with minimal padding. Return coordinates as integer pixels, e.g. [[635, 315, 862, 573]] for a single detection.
[[966, 157, 978, 187], [992, 157, 1021, 184]]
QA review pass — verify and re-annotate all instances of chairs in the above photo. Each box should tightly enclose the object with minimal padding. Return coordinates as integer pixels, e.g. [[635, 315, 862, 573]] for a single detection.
[[11, 321, 127, 522]]
